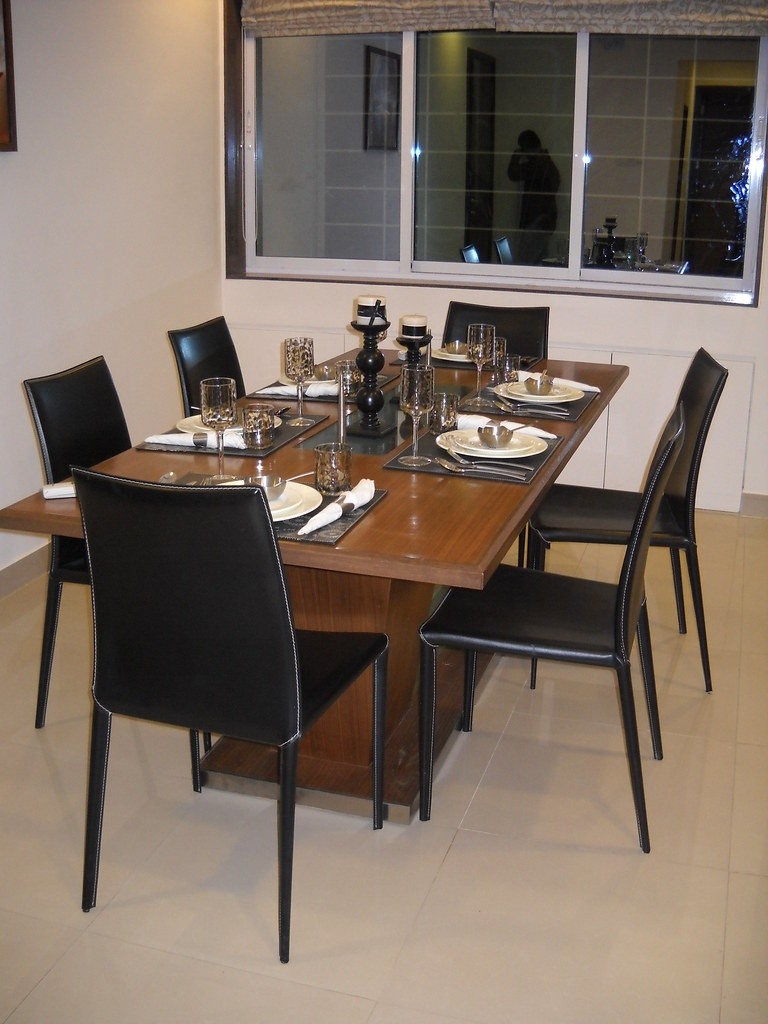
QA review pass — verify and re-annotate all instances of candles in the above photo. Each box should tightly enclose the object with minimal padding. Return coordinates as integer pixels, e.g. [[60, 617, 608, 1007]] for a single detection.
[[605, 214, 618, 224], [400, 313, 428, 340], [355, 295, 386, 325]]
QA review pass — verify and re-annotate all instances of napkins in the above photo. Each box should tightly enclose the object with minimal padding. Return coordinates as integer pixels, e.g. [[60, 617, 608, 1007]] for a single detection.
[[255, 382, 340, 397], [508, 370, 601, 393], [145, 431, 248, 450], [299, 478, 376, 535], [455, 413, 557, 439], [397, 345, 428, 361]]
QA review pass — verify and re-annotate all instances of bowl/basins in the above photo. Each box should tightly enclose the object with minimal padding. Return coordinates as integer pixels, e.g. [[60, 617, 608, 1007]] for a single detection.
[[313, 364, 338, 381], [245, 475, 286, 499], [478, 426, 513, 447], [445, 340, 466, 355], [524, 377, 552, 394]]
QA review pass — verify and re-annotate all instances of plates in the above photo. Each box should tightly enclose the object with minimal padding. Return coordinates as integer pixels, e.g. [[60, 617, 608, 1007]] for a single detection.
[[217, 479, 323, 524], [432, 349, 473, 364], [278, 372, 335, 385], [176, 415, 282, 433], [494, 382, 586, 406], [436, 430, 548, 455]]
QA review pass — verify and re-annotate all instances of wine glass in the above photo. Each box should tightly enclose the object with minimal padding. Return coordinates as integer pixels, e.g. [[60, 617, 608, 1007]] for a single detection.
[[626, 231, 648, 269], [285, 338, 316, 427], [465, 324, 505, 408], [397, 364, 434, 467], [200, 378, 236, 480]]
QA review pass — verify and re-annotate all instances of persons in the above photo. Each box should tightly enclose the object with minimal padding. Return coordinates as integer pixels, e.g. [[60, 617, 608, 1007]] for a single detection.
[[507, 129, 562, 265]]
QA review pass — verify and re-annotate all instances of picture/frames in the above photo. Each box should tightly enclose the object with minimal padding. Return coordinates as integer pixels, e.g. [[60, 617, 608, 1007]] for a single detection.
[[0, 0, 19, 153], [361, 44, 402, 151]]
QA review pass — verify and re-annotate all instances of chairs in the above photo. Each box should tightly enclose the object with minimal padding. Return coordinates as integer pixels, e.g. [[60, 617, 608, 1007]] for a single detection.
[[459, 243, 480, 263], [417, 398, 691, 855], [592, 237, 646, 263], [526, 349, 730, 692], [23, 354, 213, 755], [716, 254, 743, 277], [494, 235, 514, 265], [71, 464, 385, 963], [167, 315, 246, 417], [440, 299, 550, 361]]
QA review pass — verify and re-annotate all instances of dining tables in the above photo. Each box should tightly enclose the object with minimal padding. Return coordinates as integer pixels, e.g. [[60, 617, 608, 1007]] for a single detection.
[[532, 256, 688, 275], [0, 347, 629, 818]]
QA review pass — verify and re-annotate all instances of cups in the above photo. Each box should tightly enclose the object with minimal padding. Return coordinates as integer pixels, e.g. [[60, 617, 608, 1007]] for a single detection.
[[242, 404, 275, 451], [496, 353, 521, 385], [429, 393, 459, 436], [314, 442, 353, 497], [336, 361, 362, 398]]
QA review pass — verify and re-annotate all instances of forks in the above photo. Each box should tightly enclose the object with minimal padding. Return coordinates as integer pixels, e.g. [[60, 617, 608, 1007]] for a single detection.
[[446, 447, 535, 472], [198, 479, 212, 485], [497, 393, 567, 412]]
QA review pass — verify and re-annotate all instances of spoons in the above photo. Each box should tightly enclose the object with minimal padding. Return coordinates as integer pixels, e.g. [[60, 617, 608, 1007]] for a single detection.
[[159, 472, 177, 484], [435, 455, 526, 482], [492, 399, 570, 417], [274, 407, 291, 416]]
